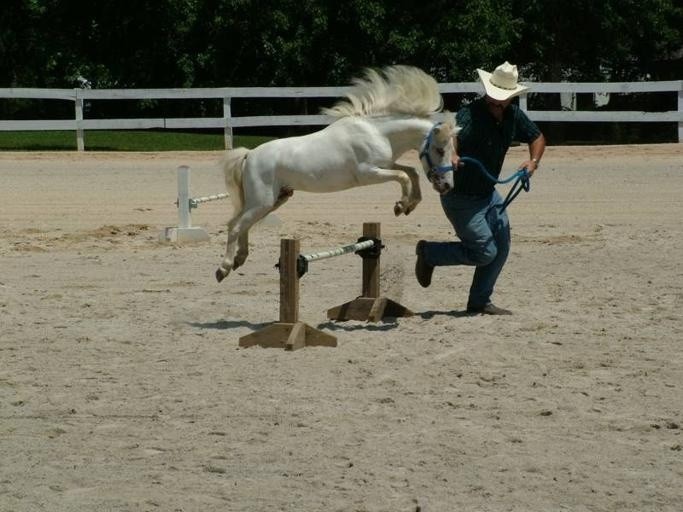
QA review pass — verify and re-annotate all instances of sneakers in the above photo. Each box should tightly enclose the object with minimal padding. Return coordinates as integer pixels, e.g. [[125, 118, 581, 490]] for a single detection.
[[415, 239, 434, 289], [468, 300, 513, 317]]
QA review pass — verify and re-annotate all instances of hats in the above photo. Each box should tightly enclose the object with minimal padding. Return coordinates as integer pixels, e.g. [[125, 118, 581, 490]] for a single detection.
[[476, 61, 530, 102]]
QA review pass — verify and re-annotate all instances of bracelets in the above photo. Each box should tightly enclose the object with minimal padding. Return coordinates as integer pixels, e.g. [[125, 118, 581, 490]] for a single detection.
[[530, 159, 539, 169]]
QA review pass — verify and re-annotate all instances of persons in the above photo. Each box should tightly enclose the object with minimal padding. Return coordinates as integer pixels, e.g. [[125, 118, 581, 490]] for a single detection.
[[415, 82, 546, 316]]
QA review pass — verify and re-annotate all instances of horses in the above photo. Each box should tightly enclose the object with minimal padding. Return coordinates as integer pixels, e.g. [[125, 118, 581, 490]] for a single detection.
[[216, 64, 463, 284]]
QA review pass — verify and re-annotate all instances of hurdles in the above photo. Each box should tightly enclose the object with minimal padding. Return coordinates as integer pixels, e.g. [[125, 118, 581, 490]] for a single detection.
[[163, 166, 230, 244], [238, 221, 415, 353]]
